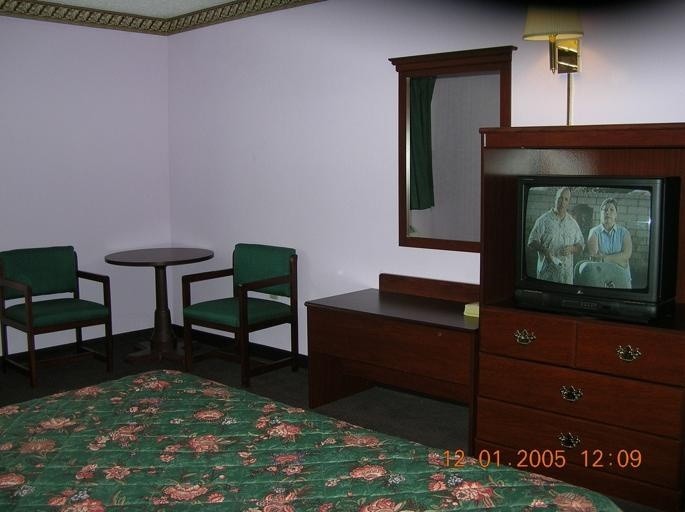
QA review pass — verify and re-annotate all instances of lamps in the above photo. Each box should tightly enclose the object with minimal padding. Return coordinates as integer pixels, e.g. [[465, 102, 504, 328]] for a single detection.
[[522, 0, 584, 76]]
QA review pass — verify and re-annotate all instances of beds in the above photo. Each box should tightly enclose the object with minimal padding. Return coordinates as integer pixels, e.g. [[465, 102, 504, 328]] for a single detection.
[[0, 367, 624, 512]]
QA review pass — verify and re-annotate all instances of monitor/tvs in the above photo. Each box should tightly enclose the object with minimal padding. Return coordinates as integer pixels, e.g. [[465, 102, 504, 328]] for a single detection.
[[514, 175, 681, 325]]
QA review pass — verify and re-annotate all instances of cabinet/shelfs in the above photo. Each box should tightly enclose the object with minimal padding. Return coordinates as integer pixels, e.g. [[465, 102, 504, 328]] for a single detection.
[[472, 122, 685, 511]]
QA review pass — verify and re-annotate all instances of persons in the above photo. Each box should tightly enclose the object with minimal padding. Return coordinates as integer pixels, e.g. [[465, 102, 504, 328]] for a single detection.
[[584, 197, 635, 288], [525, 187, 587, 286]]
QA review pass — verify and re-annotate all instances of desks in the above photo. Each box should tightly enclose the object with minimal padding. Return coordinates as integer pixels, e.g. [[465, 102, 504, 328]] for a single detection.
[[304, 273, 481, 458], [105, 248, 214, 363]]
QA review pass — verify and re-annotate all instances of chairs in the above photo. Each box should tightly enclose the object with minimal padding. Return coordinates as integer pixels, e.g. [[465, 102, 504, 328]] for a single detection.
[[181, 243, 298, 388], [0, 245, 113, 386]]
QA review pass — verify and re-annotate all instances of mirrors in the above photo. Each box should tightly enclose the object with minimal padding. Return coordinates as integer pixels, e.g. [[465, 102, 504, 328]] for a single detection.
[[389, 45, 518, 253]]
